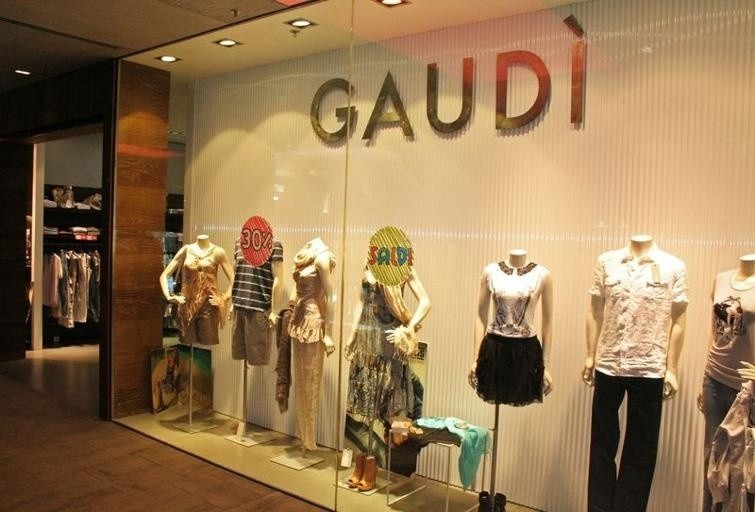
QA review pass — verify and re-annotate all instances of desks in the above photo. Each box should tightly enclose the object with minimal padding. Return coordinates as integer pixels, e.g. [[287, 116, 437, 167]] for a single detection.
[[380, 424, 491, 512]]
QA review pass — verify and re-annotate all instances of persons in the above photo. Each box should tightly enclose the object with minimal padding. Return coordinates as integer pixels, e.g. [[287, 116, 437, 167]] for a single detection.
[[468, 249, 554, 407], [277, 237, 336, 450], [228, 237, 284, 365], [697, 255, 755, 512], [159, 234, 234, 346], [344, 261, 430, 416], [582, 235, 689, 512]]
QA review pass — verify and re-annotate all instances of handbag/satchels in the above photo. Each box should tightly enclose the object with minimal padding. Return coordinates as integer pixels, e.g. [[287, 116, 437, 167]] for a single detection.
[[52, 183, 102, 211], [164, 291, 181, 329]]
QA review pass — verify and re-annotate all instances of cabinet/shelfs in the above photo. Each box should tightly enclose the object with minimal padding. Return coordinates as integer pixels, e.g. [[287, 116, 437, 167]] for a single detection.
[[41, 184, 102, 245]]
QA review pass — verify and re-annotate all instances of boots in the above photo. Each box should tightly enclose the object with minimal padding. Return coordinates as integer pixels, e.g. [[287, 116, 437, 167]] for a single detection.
[[347, 453, 376, 492], [478, 490, 507, 511]]
